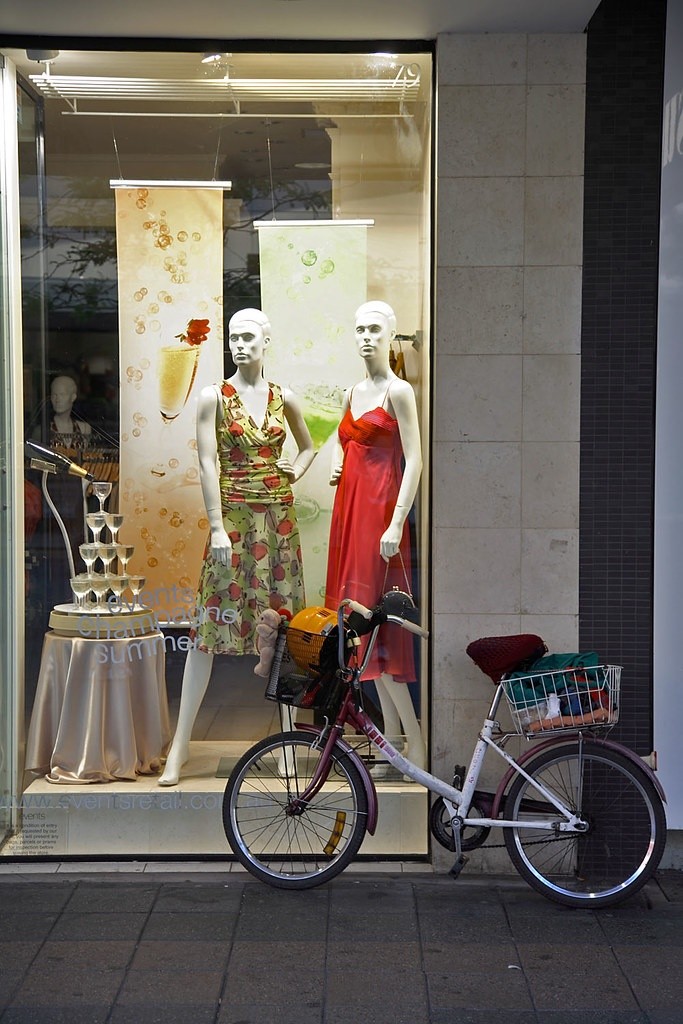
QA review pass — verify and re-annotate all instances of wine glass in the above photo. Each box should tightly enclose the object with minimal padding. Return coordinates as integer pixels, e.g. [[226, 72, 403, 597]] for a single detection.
[[69, 483, 146, 612]]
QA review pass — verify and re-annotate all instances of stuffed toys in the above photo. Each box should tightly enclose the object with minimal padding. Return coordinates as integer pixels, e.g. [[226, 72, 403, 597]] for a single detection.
[[254, 608, 295, 679]]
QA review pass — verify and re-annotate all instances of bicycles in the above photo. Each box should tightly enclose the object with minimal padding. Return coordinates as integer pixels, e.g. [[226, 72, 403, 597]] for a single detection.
[[220, 584, 669, 911]]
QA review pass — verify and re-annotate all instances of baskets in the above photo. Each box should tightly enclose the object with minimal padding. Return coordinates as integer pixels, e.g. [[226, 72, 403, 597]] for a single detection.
[[265, 625, 349, 710], [501, 665, 624, 738]]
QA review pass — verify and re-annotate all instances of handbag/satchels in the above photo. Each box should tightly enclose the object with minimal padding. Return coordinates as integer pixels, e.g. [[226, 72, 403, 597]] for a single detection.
[[380, 549, 416, 620]]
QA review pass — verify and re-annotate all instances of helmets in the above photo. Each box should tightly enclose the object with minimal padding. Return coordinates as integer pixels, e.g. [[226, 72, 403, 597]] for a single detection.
[[286, 605, 352, 676]]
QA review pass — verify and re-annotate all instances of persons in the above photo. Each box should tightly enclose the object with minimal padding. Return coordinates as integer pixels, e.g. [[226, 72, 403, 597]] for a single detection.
[[28, 377, 94, 453], [157, 307, 319, 787], [322, 301, 428, 783]]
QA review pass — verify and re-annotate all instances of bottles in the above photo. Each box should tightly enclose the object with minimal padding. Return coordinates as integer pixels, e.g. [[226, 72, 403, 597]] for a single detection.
[[23, 439, 95, 480]]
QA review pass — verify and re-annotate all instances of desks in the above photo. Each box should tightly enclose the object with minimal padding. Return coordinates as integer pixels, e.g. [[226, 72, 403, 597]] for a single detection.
[[23, 630, 173, 785]]
